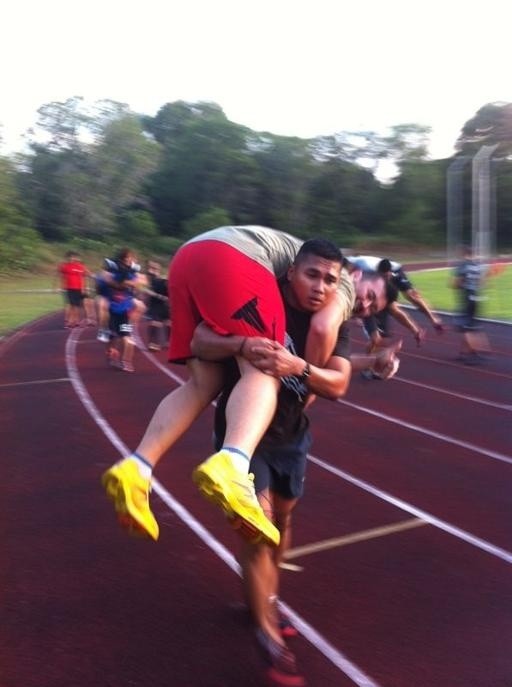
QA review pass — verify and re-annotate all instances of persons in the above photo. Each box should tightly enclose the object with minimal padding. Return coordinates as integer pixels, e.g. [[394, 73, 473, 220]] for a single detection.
[[190, 237, 354, 675], [100, 224, 399, 547]]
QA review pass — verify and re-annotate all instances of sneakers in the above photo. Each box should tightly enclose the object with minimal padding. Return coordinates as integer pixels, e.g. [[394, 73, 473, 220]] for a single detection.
[[239, 591, 302, 685], [191, 450, 281, 547], [100, 457, 160, 544]]
